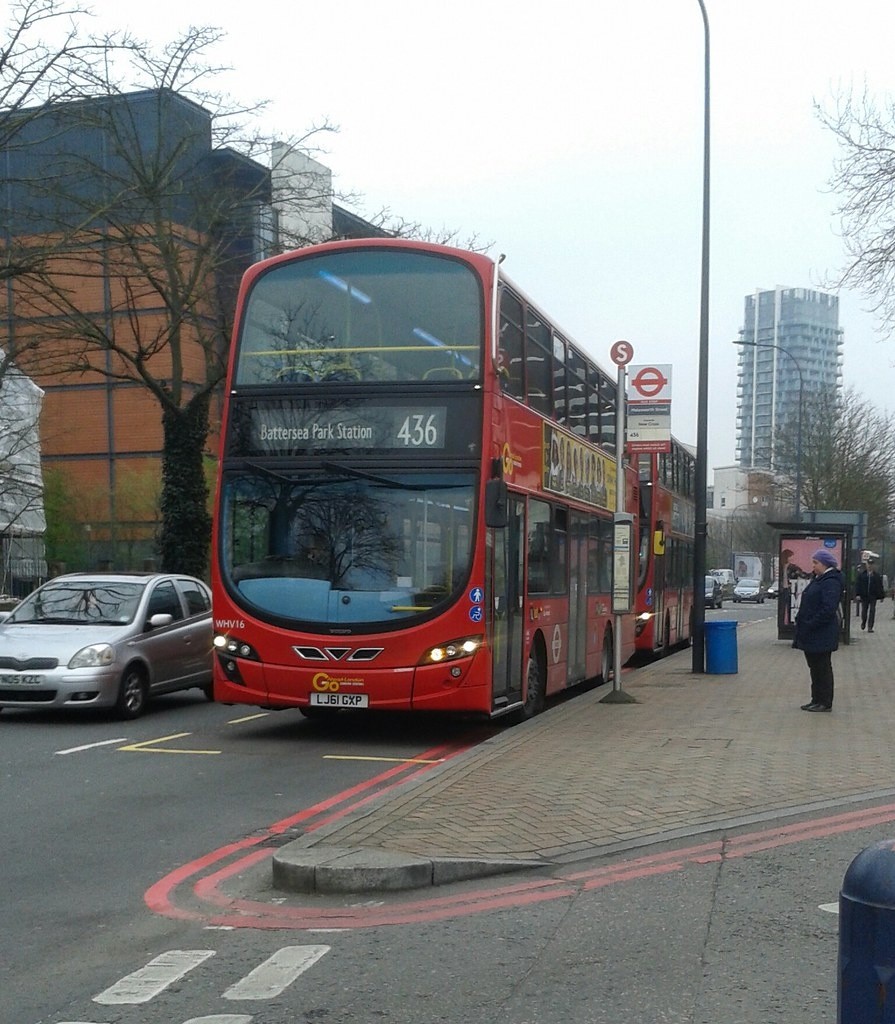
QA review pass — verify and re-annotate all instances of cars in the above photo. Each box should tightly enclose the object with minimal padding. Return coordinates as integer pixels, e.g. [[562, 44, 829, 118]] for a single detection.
[[767, 580, 779, 600], [705, 575, 723, 609], [732, 578, 766, 604], [0, 570, 213, 723]]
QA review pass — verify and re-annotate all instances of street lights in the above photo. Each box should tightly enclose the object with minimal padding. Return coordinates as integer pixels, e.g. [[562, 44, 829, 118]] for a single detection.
[[730, 339, 802, 522]]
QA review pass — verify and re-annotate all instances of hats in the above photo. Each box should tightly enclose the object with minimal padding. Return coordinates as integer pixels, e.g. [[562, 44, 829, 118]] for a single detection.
[[867, 558, 874, 563], [812, 550, 837, 567]]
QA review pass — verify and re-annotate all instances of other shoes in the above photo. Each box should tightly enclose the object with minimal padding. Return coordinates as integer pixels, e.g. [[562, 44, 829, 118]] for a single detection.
[[868, 630, 874, 632], [861, 621, 866, 629]]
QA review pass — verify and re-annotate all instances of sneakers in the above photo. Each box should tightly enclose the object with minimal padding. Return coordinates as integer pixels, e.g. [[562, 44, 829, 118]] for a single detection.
[[801, 702, 815, 710], [807, 702, 832, 712]]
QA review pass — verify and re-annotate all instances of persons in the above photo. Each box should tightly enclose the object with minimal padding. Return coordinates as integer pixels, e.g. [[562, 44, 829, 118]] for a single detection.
[[855, 558, 884, 632], [791, 549, 846, 712], [779, 549, 817, 627]]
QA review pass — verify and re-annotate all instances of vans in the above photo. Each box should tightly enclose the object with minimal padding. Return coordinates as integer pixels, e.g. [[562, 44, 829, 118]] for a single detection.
[[709, 567, 737, 584]]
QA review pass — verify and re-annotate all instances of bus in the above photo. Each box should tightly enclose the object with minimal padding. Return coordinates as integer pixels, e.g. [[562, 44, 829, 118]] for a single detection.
[[211, 239, 694, 728]]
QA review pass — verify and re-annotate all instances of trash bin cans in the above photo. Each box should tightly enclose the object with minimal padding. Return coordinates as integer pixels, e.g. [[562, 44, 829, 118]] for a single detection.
[[703, 619, 739, 674], [832, 842, 895, 1024]]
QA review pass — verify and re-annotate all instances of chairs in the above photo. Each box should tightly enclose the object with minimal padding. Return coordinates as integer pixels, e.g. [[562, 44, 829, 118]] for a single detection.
[[274, 366, 315, 383], [528, 388, 545, 411], [321, 365, 359, 383], [421, 368, 463, 380], [467, 367, 511, 391], [509, 377, 522, 398]]
